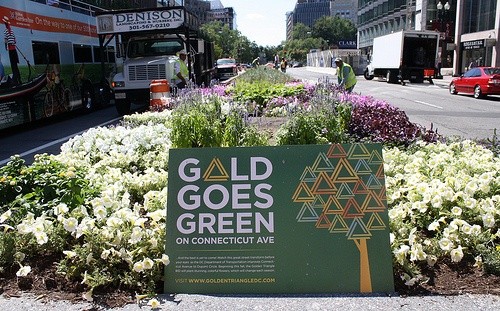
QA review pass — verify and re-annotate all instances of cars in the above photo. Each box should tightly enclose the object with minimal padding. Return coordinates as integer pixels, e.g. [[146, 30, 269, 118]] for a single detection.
[[293, 62, 303, 68], [448, 66, 500, 99], [237, 62, 251, 71]]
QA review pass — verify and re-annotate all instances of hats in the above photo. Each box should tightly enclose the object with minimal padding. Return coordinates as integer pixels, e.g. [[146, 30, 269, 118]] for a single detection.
[[2, 16, 10, 24], [179, 50, 187, 55], [334, 57, 342, 62]]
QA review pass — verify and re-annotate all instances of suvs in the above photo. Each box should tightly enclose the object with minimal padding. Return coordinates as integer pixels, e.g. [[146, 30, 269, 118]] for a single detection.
[[216, 58, 238, 81]]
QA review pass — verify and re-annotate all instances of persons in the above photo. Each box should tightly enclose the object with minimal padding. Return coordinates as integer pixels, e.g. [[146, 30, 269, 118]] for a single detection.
[[46, 64, 64, 108], [274, 53, 279, 71], [252, 57, 260, 69], [174, 50, 190, 89], [280, 57, 288, 73], [76, 64, 87, 106], [4, 22, 23, 86], [334, 57, 357, 93]]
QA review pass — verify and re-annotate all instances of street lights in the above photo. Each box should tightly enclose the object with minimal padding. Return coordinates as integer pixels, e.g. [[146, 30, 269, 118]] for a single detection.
[[436, 1, 451, 31]]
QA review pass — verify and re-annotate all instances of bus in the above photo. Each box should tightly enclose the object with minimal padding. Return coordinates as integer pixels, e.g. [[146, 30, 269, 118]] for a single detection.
[[0, 0, 125, 135]]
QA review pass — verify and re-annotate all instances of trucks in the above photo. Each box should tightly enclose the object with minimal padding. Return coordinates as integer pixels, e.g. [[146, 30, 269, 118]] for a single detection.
[[364, 30, 441, 83], [93, 5, 217, 115]]
